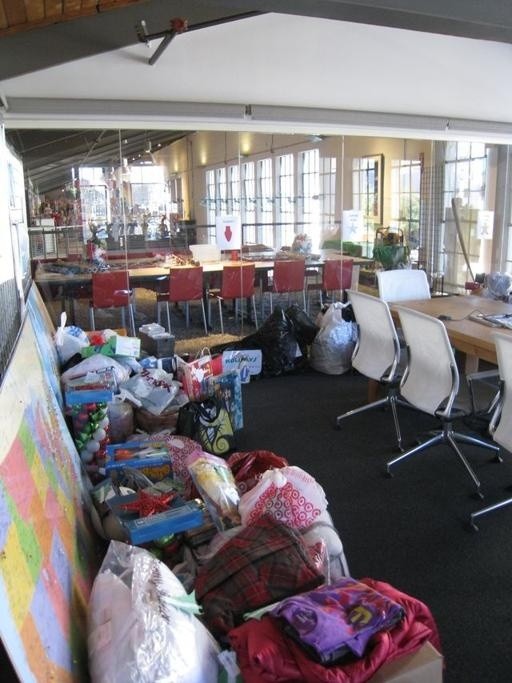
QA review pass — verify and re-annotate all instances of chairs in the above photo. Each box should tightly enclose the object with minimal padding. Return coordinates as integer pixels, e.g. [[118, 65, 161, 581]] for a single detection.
[[89, 271, 134, 334], [386, 305, 503, 501], [208, 265, 260, 332], [378, 270, 431, 304], [263, 258, 308, 326], [188, 243, 221, 262], [157, 266, 207, 332], [309, 258, 354, 314], [467, 332, 512, 529], [89, 258, 353, 335], [336, 289, 428, 454]]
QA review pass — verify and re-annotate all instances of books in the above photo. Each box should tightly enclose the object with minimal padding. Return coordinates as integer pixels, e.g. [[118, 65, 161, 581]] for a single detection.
[[469, 312, 512, 329]]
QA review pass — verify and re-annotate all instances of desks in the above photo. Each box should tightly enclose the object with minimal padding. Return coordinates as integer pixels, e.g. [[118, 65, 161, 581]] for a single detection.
[[386, 294, 512, 365], [33, 252, 374, 332]]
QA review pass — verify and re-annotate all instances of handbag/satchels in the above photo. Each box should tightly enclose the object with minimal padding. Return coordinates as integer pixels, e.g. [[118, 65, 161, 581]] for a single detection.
[[177, 398, 236, 455], [184, 347, 222, 400]]
